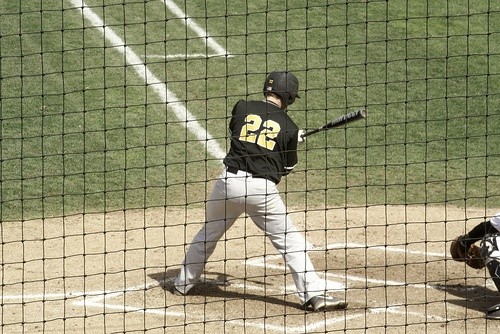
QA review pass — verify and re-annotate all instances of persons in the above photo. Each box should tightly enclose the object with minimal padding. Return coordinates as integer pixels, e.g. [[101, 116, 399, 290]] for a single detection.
[[449, 213, 500, 321], [172, 69, 348, 311]]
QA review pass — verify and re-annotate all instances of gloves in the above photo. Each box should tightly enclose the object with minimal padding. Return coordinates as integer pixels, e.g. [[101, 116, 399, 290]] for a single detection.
[[297, 130, 308, 142]]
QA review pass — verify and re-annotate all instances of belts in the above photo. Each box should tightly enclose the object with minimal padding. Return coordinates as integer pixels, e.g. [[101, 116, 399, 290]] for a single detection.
[[226, 166, 265, 178]]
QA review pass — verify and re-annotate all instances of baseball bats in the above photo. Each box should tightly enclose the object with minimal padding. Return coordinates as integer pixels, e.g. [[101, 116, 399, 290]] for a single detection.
[[302, 109, 369, 138]]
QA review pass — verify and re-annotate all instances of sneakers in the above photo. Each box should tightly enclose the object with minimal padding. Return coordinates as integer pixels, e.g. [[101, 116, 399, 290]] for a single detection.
[[486, 303, 500, 320], [309, 293, 348, 311], [174, 278, 205, 295]]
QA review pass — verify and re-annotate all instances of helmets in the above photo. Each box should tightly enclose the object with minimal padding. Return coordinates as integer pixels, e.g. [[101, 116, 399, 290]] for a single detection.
[[263, 71, 300, 106]]
